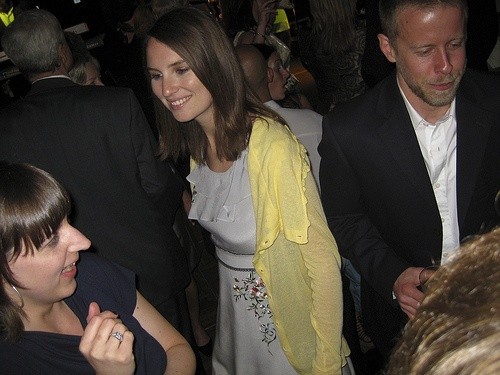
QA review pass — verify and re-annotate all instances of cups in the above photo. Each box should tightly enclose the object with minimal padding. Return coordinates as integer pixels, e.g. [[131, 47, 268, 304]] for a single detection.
[[418, 267, 436, 290]]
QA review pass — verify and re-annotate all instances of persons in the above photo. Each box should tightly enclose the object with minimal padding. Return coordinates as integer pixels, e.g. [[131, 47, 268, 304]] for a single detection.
[[141, 7, 354, 375], [216, 0, 500, 375], [0, 0, 208, 375]]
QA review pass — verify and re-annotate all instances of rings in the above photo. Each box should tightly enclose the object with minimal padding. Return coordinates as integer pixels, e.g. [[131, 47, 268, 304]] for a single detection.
[[112, 332, 122, 340]]
